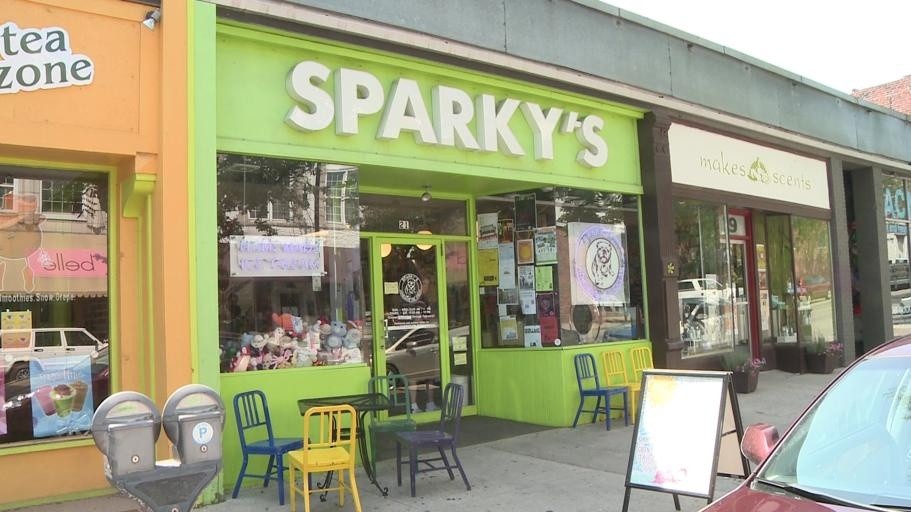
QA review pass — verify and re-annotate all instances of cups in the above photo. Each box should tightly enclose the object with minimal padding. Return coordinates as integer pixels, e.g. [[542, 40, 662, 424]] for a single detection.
[[50, 387, 77, 418], [35, 385, 56, 416], [71, 379, 89, 412]]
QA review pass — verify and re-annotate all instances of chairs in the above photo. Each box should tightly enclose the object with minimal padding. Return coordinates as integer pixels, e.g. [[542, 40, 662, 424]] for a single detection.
[[631, 346, 655, 426], [395, 381, 473, 499], [234, 387, 312, 505], [575, 352, 630, 428], [602, 349, 639, 426], [287, 404, 369, 512], [368, 374, 418, 480]]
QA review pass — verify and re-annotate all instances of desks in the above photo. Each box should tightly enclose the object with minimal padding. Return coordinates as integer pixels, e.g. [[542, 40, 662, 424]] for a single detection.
[[298, 389, 393, 502]]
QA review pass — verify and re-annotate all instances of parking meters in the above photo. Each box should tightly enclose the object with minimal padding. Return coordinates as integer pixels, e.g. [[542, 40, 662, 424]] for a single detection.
[[90, 385, 227, 511]]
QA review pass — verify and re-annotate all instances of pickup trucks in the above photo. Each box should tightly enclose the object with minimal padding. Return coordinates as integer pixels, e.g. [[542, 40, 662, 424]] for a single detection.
[[676, 277, 731, 301], [0, 326, 109, 384], [889, 258, 911, 324]]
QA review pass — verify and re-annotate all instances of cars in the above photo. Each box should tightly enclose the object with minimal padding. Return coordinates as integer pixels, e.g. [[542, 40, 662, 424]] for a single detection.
[[0, 330, 342, 442], [364, 325, 454, 391], [696, 334, 911, 512], [797, 273, 832, 302], [602, 296, 744, 356]]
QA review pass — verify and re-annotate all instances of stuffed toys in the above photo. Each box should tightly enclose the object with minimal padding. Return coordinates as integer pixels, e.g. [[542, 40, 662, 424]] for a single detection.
[[228, 310, 363, 371]]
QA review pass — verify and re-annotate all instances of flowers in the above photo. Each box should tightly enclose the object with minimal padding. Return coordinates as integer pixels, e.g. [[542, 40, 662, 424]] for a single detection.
[[825, 342, 848, 353], [733, 356, 768, 371]]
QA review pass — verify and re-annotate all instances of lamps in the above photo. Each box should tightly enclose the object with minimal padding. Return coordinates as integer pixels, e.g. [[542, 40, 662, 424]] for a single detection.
[[143, 10, 161, 30], [382, 244, 392, 259], [416, 230, 433, 251]]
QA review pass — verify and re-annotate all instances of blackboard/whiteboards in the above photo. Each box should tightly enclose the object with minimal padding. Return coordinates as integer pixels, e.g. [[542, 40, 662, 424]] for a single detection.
[[624, 370, 726, 499]]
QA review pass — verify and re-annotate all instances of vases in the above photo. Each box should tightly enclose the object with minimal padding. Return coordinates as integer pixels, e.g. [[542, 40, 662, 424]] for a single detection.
[[806, 352, 835, 373], [734, 370, 760, 394]]
